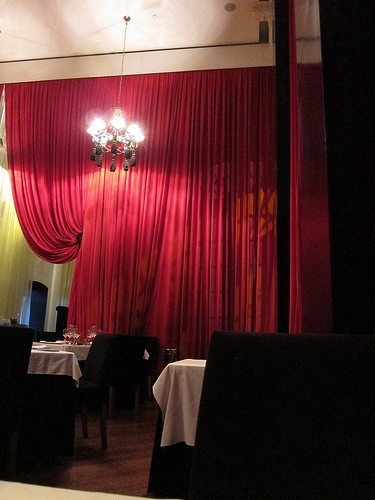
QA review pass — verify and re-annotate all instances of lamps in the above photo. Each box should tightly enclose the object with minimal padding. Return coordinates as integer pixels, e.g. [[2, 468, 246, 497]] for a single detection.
[[87, 15, 145, 172]]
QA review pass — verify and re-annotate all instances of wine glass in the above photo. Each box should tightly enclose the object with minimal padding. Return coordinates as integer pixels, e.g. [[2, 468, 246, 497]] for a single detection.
[[63, 324, 80, 345], [86, 325, 103, 345]]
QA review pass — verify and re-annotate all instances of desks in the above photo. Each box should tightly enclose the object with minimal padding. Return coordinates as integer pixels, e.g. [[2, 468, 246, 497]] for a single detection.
[[28, 340, 91, 464], [147, 357, 207, 498]]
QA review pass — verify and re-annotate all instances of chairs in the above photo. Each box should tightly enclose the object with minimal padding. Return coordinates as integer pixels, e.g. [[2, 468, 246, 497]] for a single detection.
[[190, 331, 374, 500], [0, 326, 35, 481], [129, 336, 158, 415], [77, 334, 130, 450]]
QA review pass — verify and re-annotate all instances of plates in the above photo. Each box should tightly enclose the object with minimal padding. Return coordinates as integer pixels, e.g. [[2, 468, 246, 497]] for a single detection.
[[42, 349, 59, 351]]
